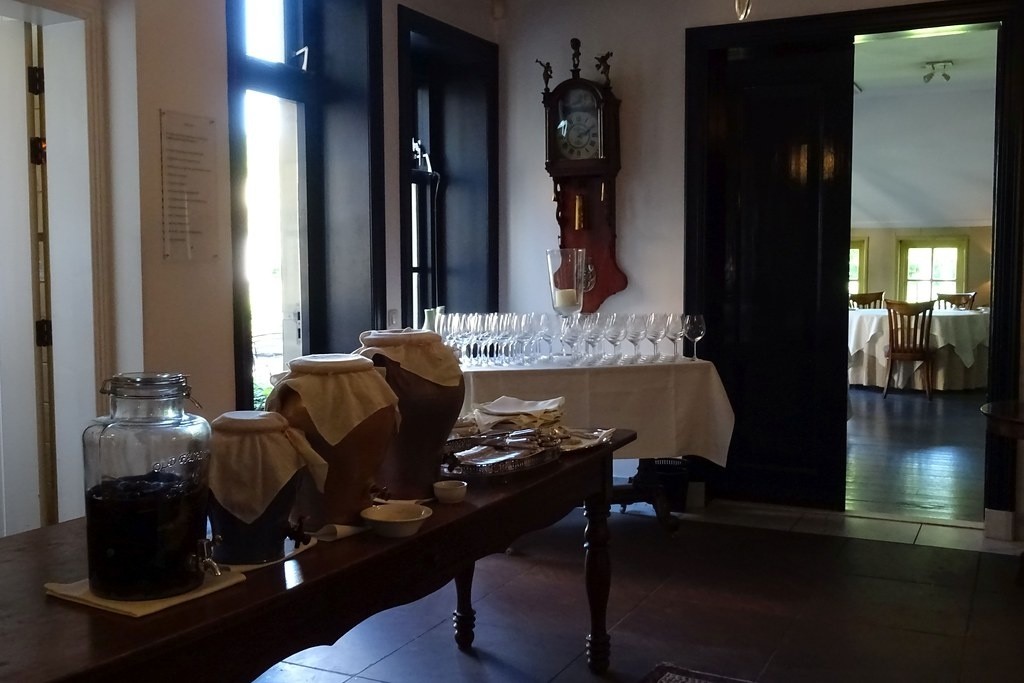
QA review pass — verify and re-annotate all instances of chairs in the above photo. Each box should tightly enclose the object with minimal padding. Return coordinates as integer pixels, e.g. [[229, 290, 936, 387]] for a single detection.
[[936, 291, 977, 310], [849, 291, 886, 310], [882, 298, 937, 401]]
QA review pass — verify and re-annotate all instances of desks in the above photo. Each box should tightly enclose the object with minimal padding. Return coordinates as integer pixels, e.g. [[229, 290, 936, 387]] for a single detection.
[[457, 353, 736, 530], [0, 425, 637, 683]]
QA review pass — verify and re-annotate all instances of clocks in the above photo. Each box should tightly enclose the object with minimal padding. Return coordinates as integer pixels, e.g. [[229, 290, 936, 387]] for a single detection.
[[531, 38, 630, 319]]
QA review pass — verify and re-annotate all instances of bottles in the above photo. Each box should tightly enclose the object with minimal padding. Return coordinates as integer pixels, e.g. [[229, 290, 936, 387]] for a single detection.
[[83, 372, 212, 602]]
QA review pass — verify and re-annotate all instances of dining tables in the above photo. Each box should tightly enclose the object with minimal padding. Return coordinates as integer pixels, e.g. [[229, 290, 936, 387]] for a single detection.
[[848, 308, 990, 391]]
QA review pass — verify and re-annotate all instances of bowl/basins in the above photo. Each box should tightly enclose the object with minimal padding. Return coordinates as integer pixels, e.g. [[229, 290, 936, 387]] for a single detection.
[[433, 480, 467, 503], [361, 504, 432, 538]]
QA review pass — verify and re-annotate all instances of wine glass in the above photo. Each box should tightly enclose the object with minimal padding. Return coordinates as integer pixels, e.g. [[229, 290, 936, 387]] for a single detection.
[[435, 249, 705, 369]]
